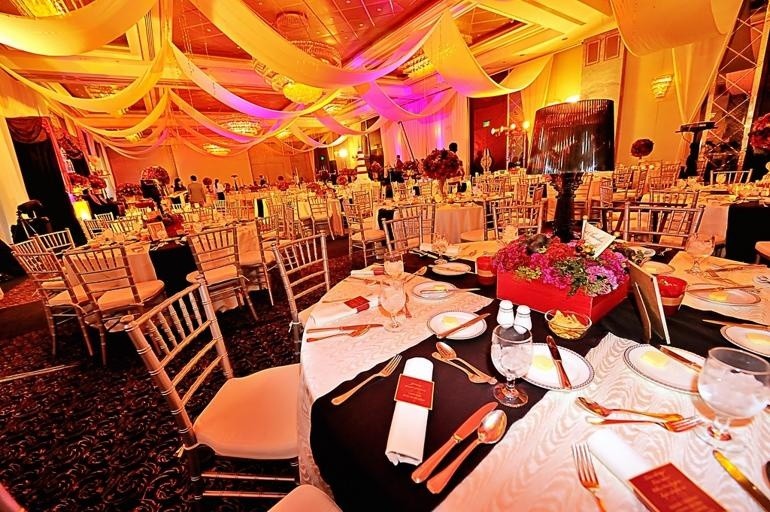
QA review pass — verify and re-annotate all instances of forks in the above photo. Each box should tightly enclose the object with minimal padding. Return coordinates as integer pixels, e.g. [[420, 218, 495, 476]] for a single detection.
[[332, 354, 402, 405], [409, 251, 428, 258], [587, 416, 704, 433], [306, 325, 369, 343], [432, 351, 489, 383], [571, 442, 609, 512]]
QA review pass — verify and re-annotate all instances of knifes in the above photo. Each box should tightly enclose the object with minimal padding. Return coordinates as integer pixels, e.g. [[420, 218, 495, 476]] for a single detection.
[[420, 287, 481, 293], [413, 247, 438, 259], [436, 312, 490, 339], [306, 324, 384, 332], [546, 334, 573, 389], [684, 285, 756, 292], [428, 264, 476, 274], [648, 338, 702, 372], [712, 450, 770, 512], [705, 263, 767, 273], [411, 399, 495, 487], [702, 319, 769, 332]]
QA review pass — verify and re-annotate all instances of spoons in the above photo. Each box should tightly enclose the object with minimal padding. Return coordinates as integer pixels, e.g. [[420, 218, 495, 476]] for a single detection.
[[431, 408, 510, 497], [436, 341, 498, 385], [577, 395, 686, 425]]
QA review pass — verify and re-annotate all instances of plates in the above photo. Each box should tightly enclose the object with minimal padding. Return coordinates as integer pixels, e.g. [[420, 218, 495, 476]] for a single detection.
[[431, 262, 471, 276], [686, 283, 761, 308], [413, 281, 457, 301], [719, 323, 770, 360], [426, 308, 488, 341], [623, 344, 706, 394], [641, 261, 675, 275], [513, 343, 594, 392]]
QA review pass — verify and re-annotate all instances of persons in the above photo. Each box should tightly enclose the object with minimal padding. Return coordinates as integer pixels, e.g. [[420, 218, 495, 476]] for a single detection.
[[172, 140, 467, 205]]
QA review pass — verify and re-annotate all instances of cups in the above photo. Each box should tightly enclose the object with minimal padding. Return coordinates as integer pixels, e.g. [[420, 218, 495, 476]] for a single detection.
[[476, 255, 497, 286], [656, 275, 688, 318]]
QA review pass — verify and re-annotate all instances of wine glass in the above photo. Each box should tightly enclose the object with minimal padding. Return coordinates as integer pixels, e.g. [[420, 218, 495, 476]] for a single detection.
[[682, 232, 718, 279], [406, 193, 444, 205], [696, 346, 770, 452], [380, 250, 405, 332], [678, 171, 770, 202], [483, 322, 533, 411]]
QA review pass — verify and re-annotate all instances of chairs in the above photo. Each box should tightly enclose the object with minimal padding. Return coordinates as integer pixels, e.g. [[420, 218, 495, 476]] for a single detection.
[[120, 273, 301, 512], [266, 484, 343, 512]]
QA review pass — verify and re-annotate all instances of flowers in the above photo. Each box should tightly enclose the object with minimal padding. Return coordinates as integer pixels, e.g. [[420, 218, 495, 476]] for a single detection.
[[630, 137, 655, 164], [421, 148, 463, 199]]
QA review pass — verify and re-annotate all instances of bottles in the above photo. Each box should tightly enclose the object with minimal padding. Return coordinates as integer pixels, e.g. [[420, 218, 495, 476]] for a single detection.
[[496, 299, 515, 330], [514, 305, 533, 334]]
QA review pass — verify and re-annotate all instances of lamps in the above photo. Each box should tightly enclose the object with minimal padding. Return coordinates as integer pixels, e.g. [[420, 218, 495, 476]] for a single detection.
[[213, 112, 260, 137], [101, 93, 129, 115], [650, 75, 675, 98], [125, 132, 143, 142], [250, 12, 343, 105], [10, 0, 84, 17], [525, 96, 616, 240], [201, 141, 232, 158]]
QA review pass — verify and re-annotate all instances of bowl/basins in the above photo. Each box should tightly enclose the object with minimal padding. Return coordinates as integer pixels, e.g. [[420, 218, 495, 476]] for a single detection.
[[544, 310, 593, 339]]
